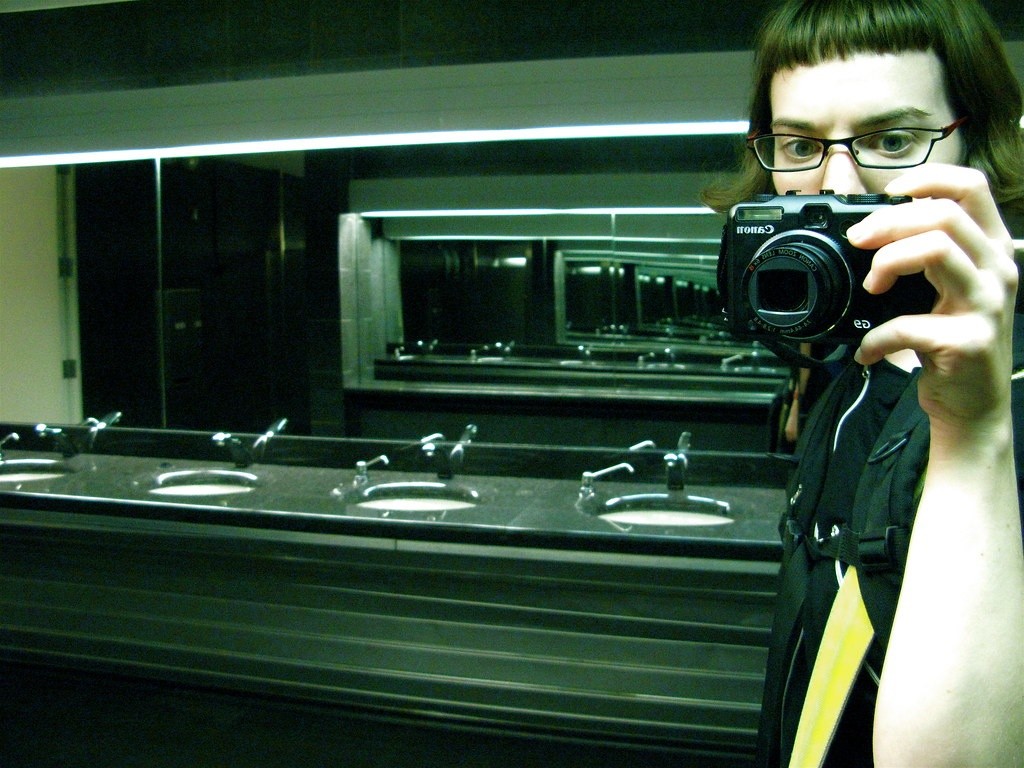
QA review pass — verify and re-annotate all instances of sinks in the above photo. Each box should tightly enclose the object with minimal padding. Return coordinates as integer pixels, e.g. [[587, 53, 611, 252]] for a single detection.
[[597, 491, 740, 525], [728, 365, 777, 376], [1, 461, 87, 482], [399, 355, 438, 360], [342, 483, 481, 511], [563, 360, 603, 367], [477, 356, 515, 362], [138, 469, 260, 494], [641, 363, 685, 371]]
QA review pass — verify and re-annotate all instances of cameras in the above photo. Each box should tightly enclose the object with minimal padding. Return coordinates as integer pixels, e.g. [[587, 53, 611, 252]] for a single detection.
[[722, 186, 942, 343]]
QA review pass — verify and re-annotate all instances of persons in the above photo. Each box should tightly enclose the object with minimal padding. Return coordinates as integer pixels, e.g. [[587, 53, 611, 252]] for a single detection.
[[698, 0, 1024, 768]]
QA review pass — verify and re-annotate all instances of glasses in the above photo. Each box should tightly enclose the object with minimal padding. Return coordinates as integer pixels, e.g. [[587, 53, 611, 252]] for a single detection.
[[747, 114, 969, 172]]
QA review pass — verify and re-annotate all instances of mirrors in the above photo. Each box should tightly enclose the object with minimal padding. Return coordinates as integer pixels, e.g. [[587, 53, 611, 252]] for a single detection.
[[0, 140, 803, 454], [359, 210, 791, 377]]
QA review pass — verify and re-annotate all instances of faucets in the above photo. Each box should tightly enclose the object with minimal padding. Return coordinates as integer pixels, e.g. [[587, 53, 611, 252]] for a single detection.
[[426, 442, 454, 480], [662, 452, 684, 491], [264, 418, 290, 435], [457, 422, 477, 442], [494, 342, 506, 355], [676, 431, 692, 451], [430, 340, 439, 350], [577, 345, 590, 364], [35, 422, 78, 457], [84, 412, 121, 427], [416, 340, 427, 354], [663, 348, 675, 363], [214, 434, 253, 467], [750, 352, 760, 365], [506, 339, 515, 353]]
